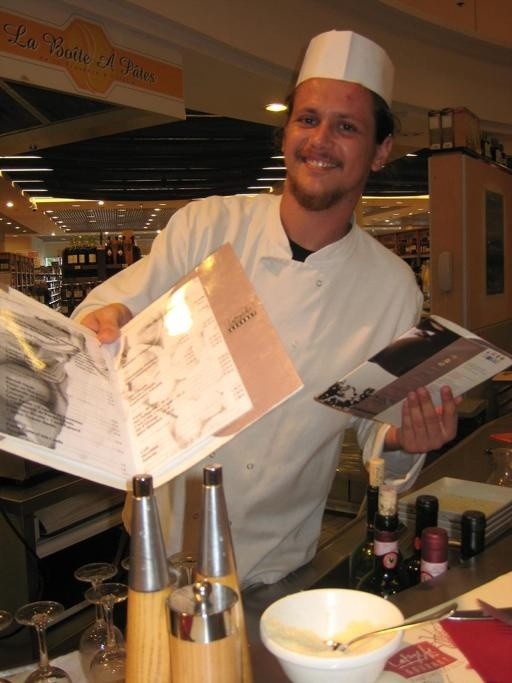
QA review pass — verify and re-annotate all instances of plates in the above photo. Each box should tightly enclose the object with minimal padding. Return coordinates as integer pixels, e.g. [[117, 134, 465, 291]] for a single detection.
[[397, 477, 512, 546]]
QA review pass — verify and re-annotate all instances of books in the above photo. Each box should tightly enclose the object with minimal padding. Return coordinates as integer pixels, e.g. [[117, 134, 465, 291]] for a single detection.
[[0, 242, 303, 495]]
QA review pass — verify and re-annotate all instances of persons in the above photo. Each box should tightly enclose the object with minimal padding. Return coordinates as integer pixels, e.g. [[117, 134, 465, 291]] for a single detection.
[[68, 77, 463, 594]]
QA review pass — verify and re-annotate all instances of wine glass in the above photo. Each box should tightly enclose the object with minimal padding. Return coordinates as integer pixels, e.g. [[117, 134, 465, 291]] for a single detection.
[[0, 549, 202, 683]]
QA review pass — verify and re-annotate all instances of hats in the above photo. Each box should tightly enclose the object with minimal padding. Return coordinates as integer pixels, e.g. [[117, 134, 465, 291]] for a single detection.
[[295, 30, 395, 110]]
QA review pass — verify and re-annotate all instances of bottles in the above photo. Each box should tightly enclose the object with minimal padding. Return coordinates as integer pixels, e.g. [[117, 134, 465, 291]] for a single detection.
[[348, 460, 486, 598], [61, 281, 103, 315], [104, 236, 141, 264], [68, 241, 97, 265]]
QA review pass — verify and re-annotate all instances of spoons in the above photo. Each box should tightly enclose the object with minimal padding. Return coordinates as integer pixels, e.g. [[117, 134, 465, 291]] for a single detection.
[[324, 602, 458, 652]]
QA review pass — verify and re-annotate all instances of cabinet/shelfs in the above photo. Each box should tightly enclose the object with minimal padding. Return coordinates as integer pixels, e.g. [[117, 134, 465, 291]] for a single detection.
[[0, 252, 35, 298], [61, 263, 130, 317], [371, 229, 430, 320], [34, 272, 62, 312]]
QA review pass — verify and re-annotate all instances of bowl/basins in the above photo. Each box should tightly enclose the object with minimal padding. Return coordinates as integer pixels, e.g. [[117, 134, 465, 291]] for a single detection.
[[258, 588, 405, 683]]
